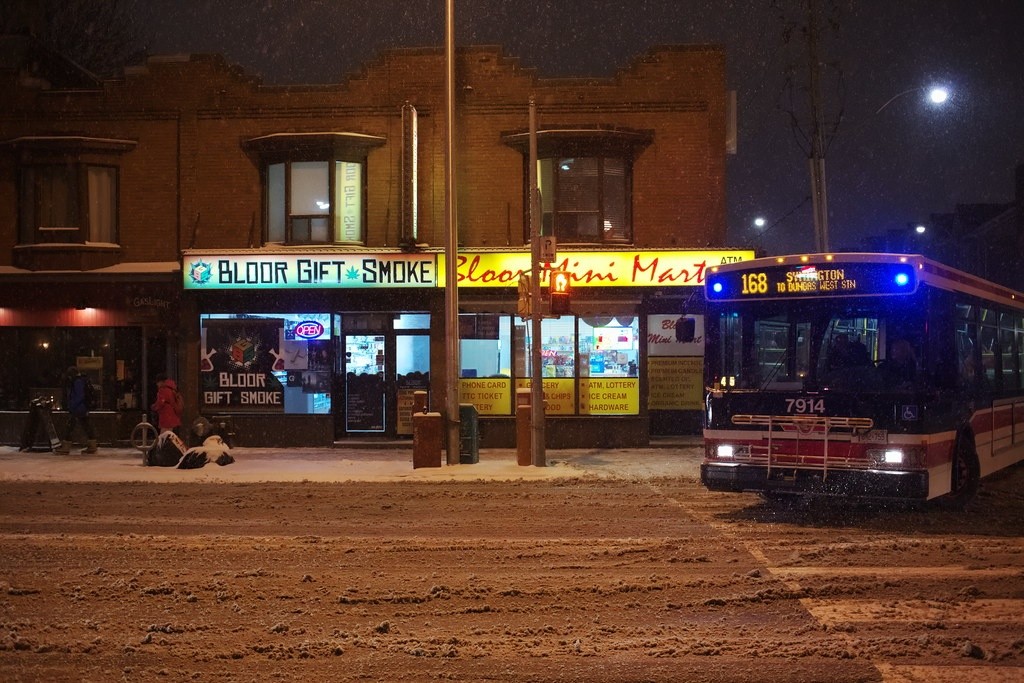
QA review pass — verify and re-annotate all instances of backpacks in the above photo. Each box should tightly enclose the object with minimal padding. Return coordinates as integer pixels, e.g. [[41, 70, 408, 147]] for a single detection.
[[158, 386, 185, 416], [78, 376, 103, 410]]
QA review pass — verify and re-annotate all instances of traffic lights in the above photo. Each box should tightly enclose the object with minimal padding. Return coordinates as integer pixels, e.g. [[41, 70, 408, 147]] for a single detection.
[[550, 271, 572, 317]]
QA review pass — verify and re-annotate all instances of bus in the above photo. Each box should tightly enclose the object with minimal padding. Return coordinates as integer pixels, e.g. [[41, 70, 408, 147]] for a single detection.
[[674, 251, 1024, 512]]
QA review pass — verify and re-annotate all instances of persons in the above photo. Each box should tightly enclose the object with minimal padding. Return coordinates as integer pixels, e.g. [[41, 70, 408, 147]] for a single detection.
[[876, 339, 919, 398], [150, 373, 182, 434], [55, 365, 99, 455]]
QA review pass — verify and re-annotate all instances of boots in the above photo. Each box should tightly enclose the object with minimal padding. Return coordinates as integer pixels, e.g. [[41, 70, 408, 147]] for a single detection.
[[51, 440, 73, 454], [81, 439, 99, 455]]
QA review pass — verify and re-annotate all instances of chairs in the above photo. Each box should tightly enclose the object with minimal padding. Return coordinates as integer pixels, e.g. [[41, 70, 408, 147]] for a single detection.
[[830, 330, 1020, 405]]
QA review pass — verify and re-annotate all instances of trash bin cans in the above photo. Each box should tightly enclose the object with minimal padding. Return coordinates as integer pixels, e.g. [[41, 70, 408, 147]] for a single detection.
[[412, 412, 443, 470], [458, 402, 480, 465], [515, 387, 545, 466]]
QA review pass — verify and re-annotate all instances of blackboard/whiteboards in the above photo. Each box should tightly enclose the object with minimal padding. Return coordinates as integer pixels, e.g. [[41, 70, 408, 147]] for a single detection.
[[19, 399, 62, 452]]
